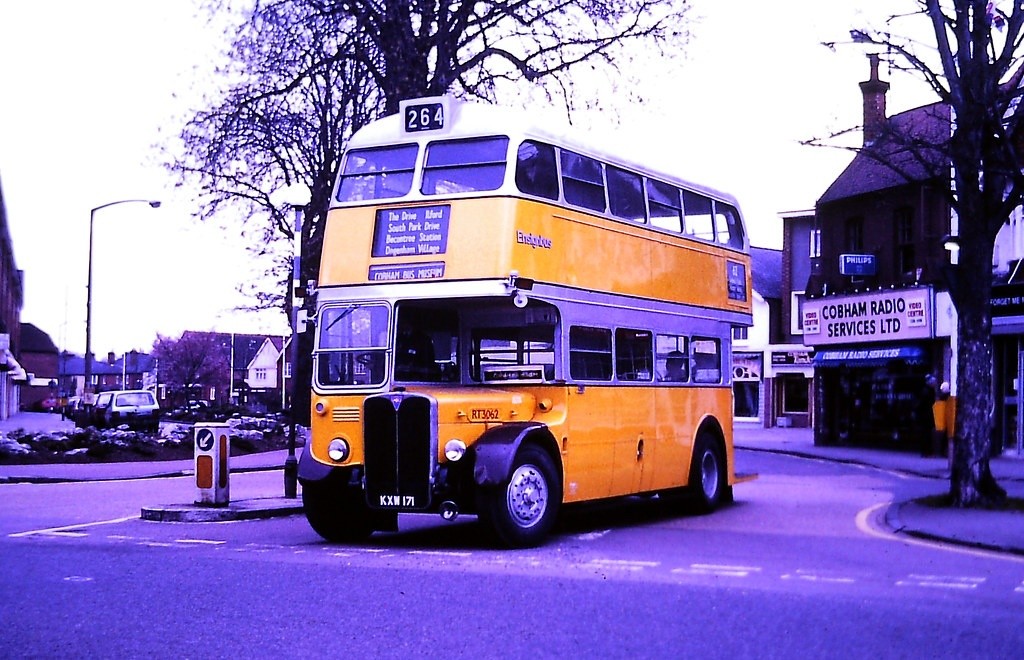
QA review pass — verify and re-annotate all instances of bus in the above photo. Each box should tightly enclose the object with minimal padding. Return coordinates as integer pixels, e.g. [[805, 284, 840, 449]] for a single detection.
[[295, 94, 753, 545]]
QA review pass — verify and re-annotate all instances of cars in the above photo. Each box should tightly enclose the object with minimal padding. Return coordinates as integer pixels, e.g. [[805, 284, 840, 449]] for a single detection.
[[35, 388, 210, 434]]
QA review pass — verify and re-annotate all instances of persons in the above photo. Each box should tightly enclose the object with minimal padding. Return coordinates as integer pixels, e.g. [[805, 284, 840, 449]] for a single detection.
[[370, 331, 422, 385], [938, 382, 950, 458], [660, 351, 687, 382], [915, 374, 937, 458]]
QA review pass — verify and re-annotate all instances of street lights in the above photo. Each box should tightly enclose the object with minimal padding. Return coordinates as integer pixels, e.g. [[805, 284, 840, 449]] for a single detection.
[[84, 198, 163, 395]]
